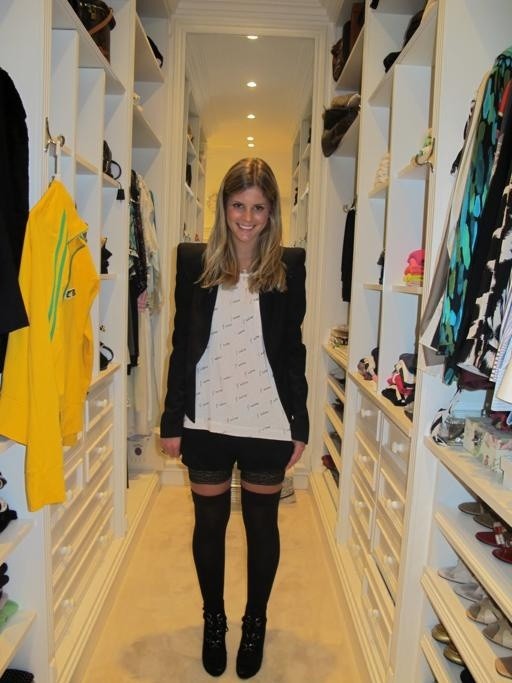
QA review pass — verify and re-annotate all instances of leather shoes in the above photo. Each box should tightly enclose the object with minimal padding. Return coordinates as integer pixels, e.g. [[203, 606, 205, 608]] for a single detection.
[[201, 606, 228, 678], [235, 608, 268, 680]]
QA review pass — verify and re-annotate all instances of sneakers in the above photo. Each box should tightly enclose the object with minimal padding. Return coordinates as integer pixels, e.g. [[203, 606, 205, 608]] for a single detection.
[[442, 644, 467, 668], [431, 621, 452, 645], [460, 667, 477, 683]]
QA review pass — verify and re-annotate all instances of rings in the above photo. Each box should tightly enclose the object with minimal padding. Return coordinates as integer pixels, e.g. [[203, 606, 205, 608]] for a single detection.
[[161, 448, 168, 455]]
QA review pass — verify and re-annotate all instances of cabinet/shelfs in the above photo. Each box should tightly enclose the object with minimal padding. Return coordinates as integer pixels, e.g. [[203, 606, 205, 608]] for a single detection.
[[0, 0, 187, 682], [304, 0, 512, 682], [291, 81, 310, 250], [182, 60, 209, 245]]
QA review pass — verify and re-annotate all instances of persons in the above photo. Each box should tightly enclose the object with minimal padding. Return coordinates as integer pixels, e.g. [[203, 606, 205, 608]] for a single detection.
[[160, 156, 308, 680]]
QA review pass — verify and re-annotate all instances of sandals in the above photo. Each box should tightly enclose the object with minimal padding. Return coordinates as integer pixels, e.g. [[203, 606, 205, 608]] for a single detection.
[[491, 547, 512, 564], [495, 655, 512, 679]]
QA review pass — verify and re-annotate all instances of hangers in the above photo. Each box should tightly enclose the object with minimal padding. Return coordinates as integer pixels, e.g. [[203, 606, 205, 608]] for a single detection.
[[45, 137, 66, 188]]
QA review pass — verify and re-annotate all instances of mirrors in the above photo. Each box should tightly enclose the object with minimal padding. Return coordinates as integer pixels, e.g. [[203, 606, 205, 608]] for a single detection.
[[161, 20, 330, 494]]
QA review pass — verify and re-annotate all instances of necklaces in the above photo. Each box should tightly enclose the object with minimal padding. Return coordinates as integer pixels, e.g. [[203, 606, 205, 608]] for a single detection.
[[238, 253, 257, 273]]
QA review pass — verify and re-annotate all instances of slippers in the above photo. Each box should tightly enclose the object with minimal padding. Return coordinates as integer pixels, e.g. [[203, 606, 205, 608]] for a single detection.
[[453, 583, 492, 602], [466, 602, 509, 624], [457, 501, 492, 515], [438, 565, 477, 584], [481, 621, 512, 650], [472, 511, 507, 529], [475, 526, 512, 548]]
